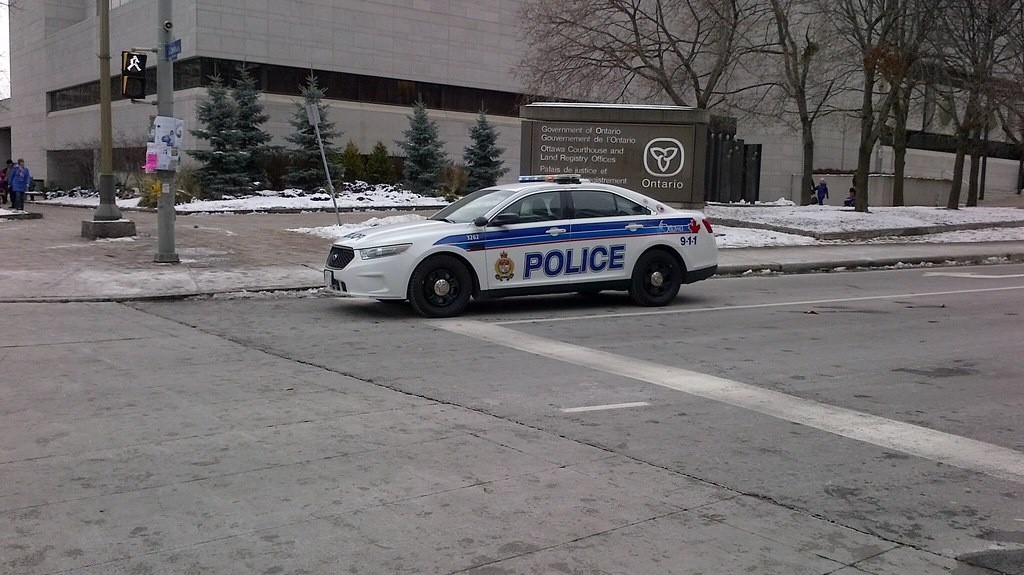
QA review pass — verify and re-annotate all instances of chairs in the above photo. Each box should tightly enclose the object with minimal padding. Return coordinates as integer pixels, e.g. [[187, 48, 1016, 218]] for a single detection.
[[530, 194, 613, 222]]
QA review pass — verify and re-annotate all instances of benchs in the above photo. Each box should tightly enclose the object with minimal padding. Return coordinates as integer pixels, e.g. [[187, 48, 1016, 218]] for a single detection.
[[24, 179, 44, 200]]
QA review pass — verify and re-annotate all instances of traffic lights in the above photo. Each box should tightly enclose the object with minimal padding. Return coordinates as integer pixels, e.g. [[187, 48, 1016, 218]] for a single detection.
[[124, 54, 148, 97]]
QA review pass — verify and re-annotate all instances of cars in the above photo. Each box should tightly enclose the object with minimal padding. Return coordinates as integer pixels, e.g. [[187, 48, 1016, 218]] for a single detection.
[[324, 173, 719, 317]]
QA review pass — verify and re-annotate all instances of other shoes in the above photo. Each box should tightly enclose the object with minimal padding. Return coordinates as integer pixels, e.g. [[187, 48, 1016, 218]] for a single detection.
[[3, 200, 8, 204], [9, 207, 23, 210]]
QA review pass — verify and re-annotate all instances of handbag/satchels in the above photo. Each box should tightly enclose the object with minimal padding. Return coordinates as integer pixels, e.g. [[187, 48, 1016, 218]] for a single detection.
[[812, 197, 818, 204]]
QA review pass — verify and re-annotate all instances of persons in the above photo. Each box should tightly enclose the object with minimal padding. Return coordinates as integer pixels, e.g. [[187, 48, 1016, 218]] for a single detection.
[[167, 130, 175, 146], [811, 175, 830, 205], [843, 169, 858, 207], [0, 158, 36, 210]]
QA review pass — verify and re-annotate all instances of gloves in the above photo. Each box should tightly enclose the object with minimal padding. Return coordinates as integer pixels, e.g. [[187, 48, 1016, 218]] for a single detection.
[[26, 184, 30, 187], [8, 184, 11, 188]]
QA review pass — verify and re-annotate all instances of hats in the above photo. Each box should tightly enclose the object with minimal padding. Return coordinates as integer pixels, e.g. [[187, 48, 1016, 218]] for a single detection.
[[820, 178, 824, 182]]
